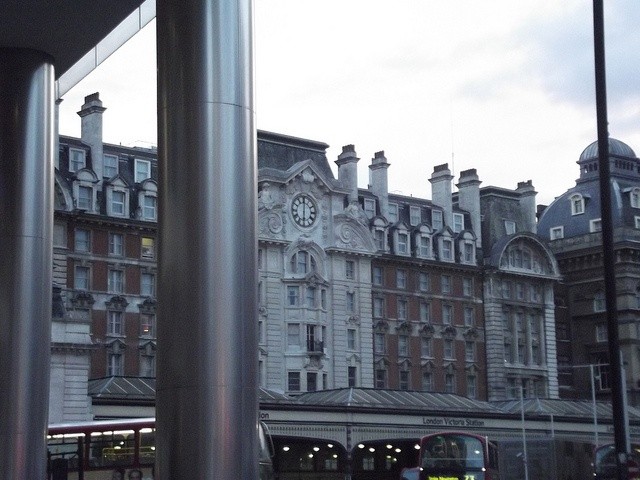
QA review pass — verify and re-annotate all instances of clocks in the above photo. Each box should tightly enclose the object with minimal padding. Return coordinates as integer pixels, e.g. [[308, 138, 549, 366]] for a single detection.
[[288, 190, 321, 232]]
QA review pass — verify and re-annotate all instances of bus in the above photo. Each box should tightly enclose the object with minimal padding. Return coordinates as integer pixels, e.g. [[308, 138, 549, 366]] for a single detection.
[[46, 416, 275, 480], [596, 443, 640, 480], [419, 432, 498, 480]]
